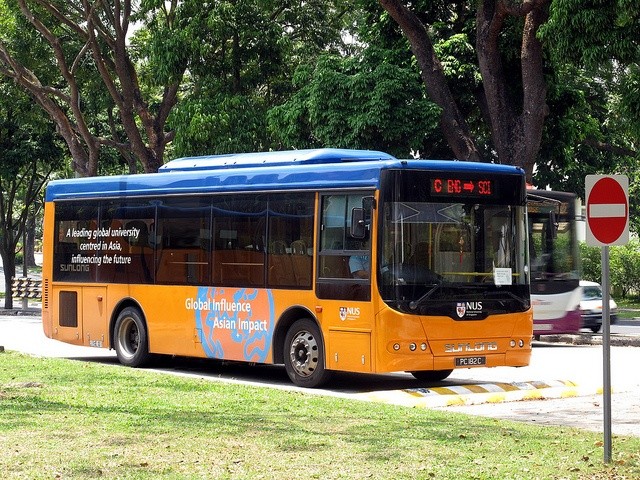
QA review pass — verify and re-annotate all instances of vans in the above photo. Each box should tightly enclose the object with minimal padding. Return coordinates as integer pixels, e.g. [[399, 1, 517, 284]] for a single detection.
[[579, 280, 618, 332]]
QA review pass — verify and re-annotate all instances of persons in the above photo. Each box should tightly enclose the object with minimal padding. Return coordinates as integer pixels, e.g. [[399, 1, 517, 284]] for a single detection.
[[408, 241, 433, 272], [348, 233, 384, 280], [390, 240, 411, 274]]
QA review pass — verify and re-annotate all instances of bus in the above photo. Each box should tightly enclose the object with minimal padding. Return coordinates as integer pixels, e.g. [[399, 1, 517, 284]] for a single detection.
[[525, 189, 586, 341], [41, 148, 534, 388]]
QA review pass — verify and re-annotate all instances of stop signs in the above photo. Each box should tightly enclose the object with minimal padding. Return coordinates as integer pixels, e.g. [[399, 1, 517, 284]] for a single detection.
[[584, 175, 629, 247]]
